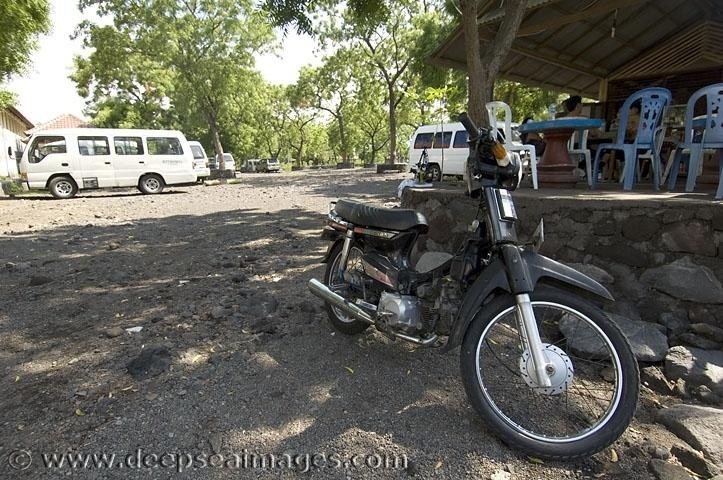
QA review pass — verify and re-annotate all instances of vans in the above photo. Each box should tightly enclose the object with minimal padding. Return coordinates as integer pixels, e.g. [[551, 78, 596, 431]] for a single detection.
[[214, 153, 236, 172], [241, 158, 280, 173], [410, 123, 521, 181], [188, 140, 210, 183]]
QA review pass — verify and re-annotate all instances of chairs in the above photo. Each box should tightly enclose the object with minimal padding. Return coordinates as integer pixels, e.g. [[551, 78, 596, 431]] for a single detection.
[[485, 83, 723, 199]]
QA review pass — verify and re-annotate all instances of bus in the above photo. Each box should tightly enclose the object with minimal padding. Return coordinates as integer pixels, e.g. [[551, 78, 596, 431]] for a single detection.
[[8, 129, 197, 199]]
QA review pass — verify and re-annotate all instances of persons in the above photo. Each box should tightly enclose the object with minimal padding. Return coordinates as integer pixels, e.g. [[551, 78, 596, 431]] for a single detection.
[[554, 100, 569, 118], [519, 117, 545, 157], [565, 95, 604, 181], [600, 107, 648, 184]]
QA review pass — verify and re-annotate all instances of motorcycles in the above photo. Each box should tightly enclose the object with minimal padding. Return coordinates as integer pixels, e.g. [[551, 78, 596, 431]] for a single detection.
[[308, 113, 640, 461]]
